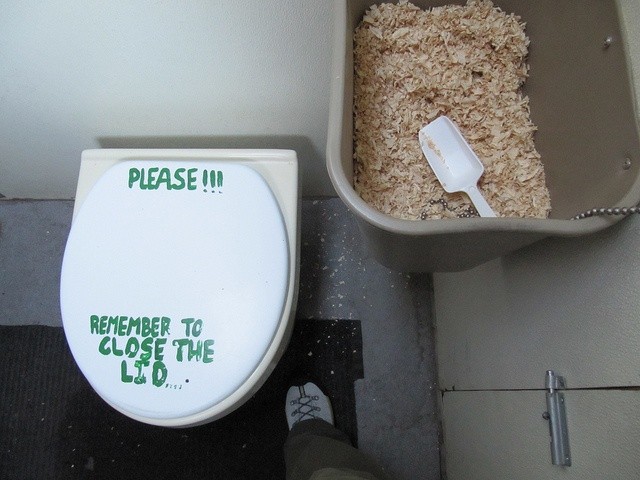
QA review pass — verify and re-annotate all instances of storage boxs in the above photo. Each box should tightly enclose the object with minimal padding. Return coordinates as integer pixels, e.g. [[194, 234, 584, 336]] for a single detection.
[[326, 0, 639, 275]]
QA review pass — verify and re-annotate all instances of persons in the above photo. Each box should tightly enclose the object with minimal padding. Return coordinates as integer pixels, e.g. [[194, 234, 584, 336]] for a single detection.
[[284, 373, 395, 480]]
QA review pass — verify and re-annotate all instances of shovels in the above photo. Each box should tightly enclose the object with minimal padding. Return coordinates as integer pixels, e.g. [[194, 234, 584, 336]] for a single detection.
[[418, 116, 496, 217]]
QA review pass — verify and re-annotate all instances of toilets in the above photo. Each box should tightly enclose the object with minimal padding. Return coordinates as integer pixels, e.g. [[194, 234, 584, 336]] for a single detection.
[[60, 149, 302, 428]]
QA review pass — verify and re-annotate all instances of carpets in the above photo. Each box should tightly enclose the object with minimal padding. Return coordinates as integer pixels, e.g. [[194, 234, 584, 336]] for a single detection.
[[0, 317, 365, 479]]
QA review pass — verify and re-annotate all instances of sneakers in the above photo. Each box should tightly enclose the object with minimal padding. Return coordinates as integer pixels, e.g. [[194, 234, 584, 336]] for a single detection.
[[284, 371, 335, 430]]
